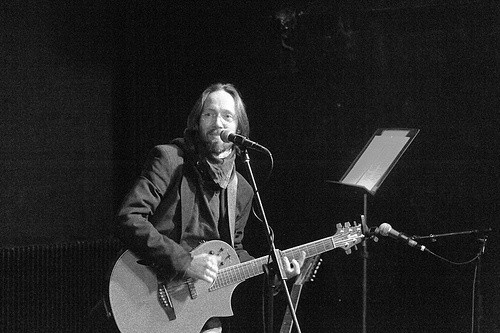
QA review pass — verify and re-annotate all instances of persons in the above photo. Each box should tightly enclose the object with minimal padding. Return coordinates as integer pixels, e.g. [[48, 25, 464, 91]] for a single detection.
[[115, 83, 306, 333]]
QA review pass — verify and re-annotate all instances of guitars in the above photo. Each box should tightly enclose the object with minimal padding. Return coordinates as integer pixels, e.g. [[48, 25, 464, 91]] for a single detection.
[[103, 220, 365, 333], [280, 254, 322, 332]]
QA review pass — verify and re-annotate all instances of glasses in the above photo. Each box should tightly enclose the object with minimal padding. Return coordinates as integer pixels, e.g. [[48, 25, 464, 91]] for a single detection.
[[200, 112, 235, 122]]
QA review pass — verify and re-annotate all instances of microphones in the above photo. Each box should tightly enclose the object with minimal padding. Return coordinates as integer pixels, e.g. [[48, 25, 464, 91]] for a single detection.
[[378, 223, 432, 254], [220, 130, 272, 156]]
